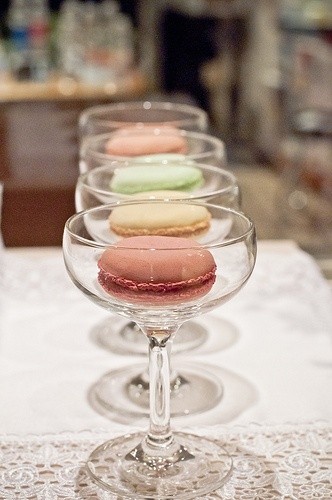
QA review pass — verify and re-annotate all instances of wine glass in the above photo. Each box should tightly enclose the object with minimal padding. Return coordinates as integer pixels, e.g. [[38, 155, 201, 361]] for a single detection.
[[72, 160, 243, 415], [76, 127, 226, 352], [62, 199, 258, 499]]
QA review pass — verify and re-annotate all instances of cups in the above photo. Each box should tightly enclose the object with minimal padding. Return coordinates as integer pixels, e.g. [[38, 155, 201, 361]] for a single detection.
[[79, 100, 208, 140]]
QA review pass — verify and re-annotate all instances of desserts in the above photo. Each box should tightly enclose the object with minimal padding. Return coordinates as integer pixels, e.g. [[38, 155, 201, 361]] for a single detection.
[[97, 126, 217, 304]]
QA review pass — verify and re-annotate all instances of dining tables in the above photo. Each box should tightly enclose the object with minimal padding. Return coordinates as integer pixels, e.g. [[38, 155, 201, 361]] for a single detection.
[[0, 236, 332, 500]]
